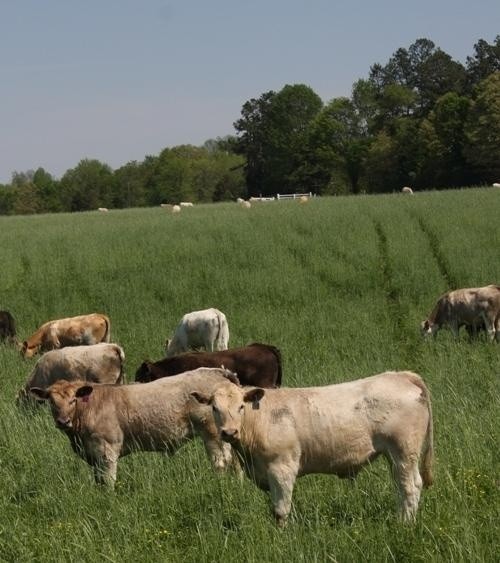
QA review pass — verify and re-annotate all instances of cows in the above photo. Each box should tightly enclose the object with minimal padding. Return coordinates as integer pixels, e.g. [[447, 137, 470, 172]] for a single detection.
[[191, 369, 434, 531], [419, 282, 500, 348], [13, 343, 126, 420], [134, 343, 283, 388], [29, 367, 244, 499], [164, 308, 231, 357], [0, 311, 18, 348], [14, 312, 111, 368]]
